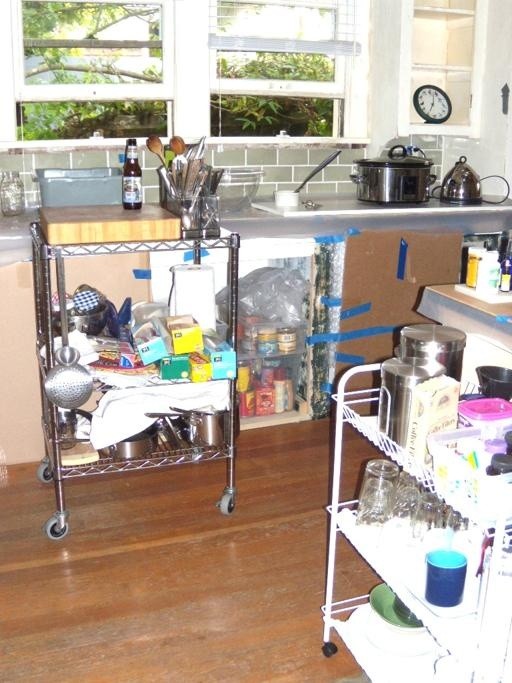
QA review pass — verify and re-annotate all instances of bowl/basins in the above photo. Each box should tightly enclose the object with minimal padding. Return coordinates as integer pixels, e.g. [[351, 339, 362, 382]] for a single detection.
[[58, 303, 109, 336], [477, 366, 512, 400], [209, 171, 262, 214]]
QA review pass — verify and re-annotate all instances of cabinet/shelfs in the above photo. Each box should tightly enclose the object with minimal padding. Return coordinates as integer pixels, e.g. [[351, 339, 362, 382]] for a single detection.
[[320, 363, 512, 683], [364, 0, 484, 161]]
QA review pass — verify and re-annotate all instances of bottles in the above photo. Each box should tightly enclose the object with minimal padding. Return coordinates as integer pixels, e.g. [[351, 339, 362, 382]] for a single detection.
[[245, 321, 298, 354], [465, 247, 512, 296], [391, 595, 421, 624], [484, 429, 511, 476], [237, 365, 296, 413], [120, 135, 144, 212], [2, 172, 25, 215], [381, 319, 464, 445]]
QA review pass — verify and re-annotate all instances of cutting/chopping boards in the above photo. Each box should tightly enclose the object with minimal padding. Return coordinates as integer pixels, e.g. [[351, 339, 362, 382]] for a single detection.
[[39, 205, 182, 244]]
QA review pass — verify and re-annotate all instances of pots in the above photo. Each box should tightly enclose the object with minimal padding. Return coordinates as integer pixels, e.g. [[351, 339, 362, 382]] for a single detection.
[[180, 409, 227, 446], [350, 146, 436, 202], [92, 424, 159, 464]]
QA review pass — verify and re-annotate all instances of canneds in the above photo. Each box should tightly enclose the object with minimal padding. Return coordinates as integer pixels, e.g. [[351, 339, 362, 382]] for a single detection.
[[260, 366, 274, 387], [235, 361, 251, 392], [239, 386, 256, 417], [237, 315, 298, 353]]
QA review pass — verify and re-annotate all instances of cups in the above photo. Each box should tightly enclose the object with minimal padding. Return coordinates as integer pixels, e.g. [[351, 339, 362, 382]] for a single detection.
[[272, 190, 300, 208], [425, 551, 465, 607], [358, 458, 465, 531]]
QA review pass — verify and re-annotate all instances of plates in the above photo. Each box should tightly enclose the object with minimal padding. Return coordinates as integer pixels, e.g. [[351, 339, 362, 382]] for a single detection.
[[369, 581, 426, 629]]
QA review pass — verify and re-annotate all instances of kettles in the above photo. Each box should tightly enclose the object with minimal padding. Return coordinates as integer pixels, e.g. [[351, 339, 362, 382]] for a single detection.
[[443, 155, 483, 203]]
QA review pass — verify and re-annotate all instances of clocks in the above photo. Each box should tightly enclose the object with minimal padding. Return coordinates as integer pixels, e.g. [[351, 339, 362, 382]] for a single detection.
[[413, 85, 452, 123]]
